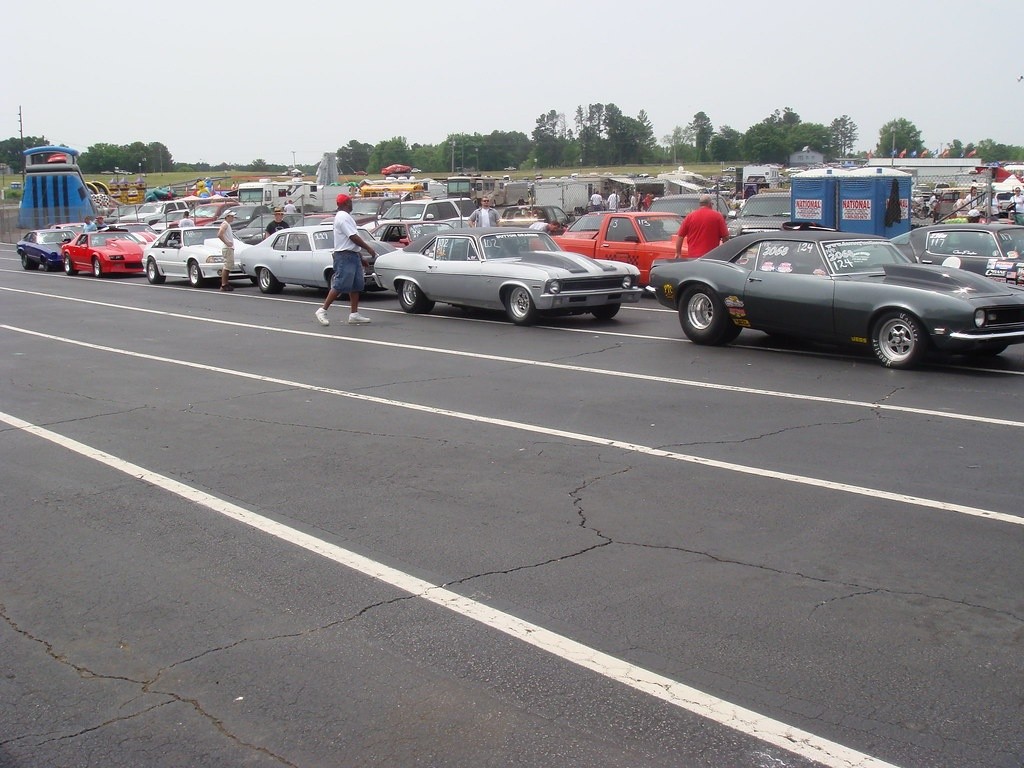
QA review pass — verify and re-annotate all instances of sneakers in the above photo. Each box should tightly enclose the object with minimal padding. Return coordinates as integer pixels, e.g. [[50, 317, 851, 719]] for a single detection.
[[315, 308, 329, 326], [348, 312, 371, 323]]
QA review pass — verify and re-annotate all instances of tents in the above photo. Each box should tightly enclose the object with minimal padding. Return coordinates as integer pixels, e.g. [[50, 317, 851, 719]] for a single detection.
[[993, 174, 1024, 193]]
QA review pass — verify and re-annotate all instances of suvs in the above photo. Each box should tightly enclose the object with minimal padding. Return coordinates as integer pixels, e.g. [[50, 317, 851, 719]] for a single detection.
[[335, 196, 477, 246]]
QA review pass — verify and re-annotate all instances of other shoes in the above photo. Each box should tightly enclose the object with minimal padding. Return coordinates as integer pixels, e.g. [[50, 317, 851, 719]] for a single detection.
[[221, 284, 234, 290]]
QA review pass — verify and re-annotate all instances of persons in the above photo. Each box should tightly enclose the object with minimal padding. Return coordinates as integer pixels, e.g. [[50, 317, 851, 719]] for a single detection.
[[315, 194, 375, 325], [910, 192, 937, 218], [588, 188, 652, 212], [284, 199, 297, 214], [517, 194, 535, 206], [529, 220, 560, 238], [264, 207, 290, 287], [468, 196, 502, 228], [217, 210, 237, 291], [965, 186, 1024, 223], [675, 194, 730, 258], [178, 211, 196, 236], [82, 217, 107, 232]]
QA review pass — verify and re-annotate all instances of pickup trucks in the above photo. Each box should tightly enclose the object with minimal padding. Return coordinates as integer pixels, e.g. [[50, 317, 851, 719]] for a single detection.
[[529, 212, 730, 297], [504, 166, 516, 171], [496, 205, 575, 236]]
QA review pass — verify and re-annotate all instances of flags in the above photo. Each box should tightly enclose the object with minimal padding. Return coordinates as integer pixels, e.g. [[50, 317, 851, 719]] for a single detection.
[[865, 148, 977, 159]]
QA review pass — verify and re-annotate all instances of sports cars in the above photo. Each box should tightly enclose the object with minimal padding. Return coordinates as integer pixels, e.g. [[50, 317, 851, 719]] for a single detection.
[[17, 221, 165, 278]]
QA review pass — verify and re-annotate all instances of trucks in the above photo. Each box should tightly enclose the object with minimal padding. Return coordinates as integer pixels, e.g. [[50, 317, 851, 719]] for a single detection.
[[911, 173, 1024, 218]]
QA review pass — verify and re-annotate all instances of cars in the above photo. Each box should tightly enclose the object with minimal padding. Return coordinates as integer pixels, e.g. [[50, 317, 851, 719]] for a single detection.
[[561, 210, 640, 239], [641, 194, 731, 221], [101, 170, 114, 175], [382, 164, 412, 175], [410, 168, 421, 173], [94, 200, 475, 301], [645, 223, 1024, 369], [371, 226, 643, 326], [356, 170, 368, 176], [727, 192, 801, 240], [708, 156, 841, 184]]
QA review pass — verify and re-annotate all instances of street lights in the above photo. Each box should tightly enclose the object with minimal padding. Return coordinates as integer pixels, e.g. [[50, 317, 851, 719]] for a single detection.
[[890, 117, 896, 167], [474, 145, 479, 177]]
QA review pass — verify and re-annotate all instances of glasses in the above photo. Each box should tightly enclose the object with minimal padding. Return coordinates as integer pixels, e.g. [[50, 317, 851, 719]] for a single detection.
[[1015, 190, 1021, 193], [483, 200, 490, 203], [276, 213, 285, 216], [710, 202, 714, 206]]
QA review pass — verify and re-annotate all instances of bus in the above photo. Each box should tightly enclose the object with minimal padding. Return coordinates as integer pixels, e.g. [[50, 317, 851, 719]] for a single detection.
[[446, 176, 506, 206], [238, 179, 317, 213], [360, 183, 422, 198]]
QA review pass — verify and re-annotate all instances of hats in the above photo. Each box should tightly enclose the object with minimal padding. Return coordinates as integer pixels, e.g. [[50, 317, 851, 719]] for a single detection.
[[222, 210, 236, 218], [336, 193, 352, 206], [272, 206, 287, 215], [96, 216, 103, 220]]
